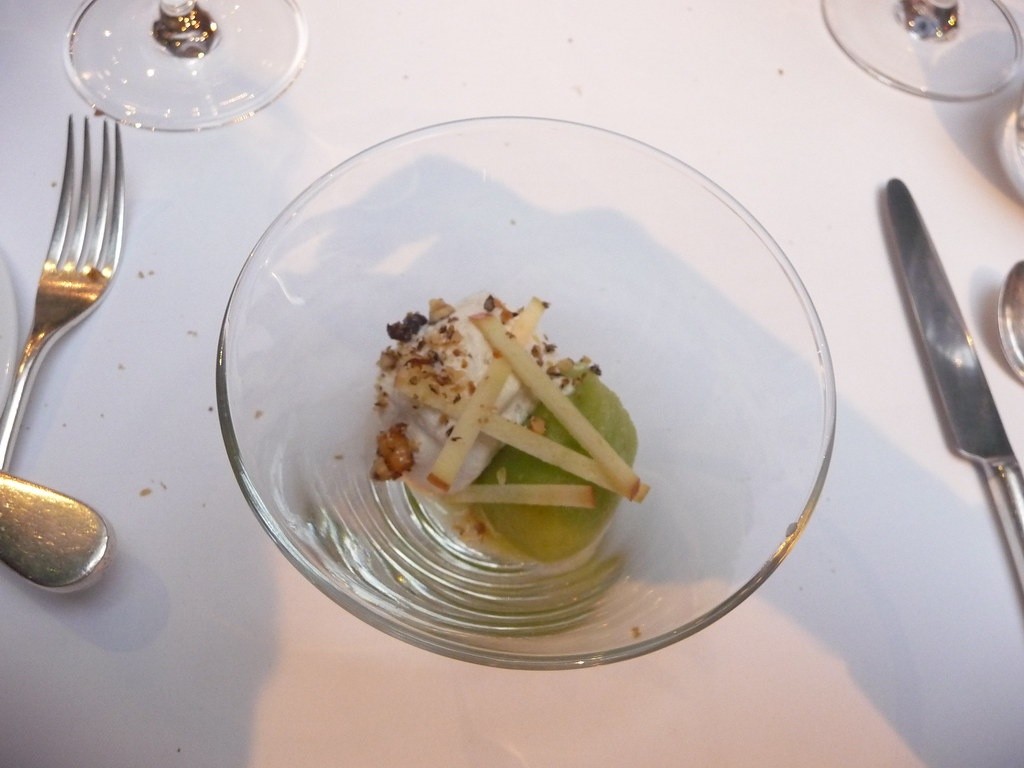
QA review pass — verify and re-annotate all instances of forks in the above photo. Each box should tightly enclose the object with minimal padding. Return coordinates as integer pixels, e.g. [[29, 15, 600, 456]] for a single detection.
[[0, 115, 125, 476]]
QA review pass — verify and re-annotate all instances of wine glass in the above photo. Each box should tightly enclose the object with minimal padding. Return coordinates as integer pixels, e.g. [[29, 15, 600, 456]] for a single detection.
[[824, 1, 1024, 102], [62, 0, 310, 132]]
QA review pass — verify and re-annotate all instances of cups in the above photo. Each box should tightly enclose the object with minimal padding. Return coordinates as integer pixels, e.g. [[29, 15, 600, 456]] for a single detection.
[[998, 104, 1024, 198]]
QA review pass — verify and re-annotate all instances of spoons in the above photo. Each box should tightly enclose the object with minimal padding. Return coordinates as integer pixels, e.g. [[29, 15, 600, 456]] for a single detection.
[[996, 261, 1024, 384]]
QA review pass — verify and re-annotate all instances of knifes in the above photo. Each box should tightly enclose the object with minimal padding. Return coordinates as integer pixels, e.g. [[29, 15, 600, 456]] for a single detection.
[[885, 178, 1024, 592]]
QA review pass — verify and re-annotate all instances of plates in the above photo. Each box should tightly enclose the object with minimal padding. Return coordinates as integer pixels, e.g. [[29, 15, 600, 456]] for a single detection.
[[217, 118, 836, 671]]
